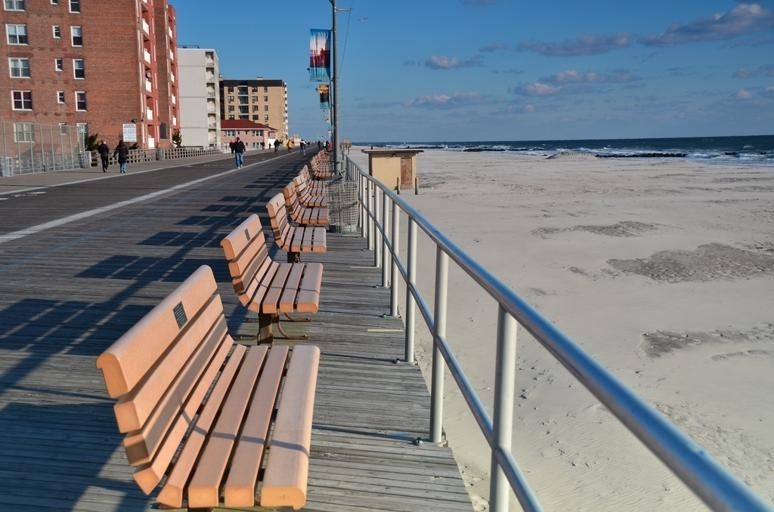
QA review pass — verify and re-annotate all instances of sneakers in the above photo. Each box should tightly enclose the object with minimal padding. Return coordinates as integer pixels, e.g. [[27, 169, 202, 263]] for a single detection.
[[103, 169, 108, 172], [120, 170, 127, 174], [237, 165, 243, 169]]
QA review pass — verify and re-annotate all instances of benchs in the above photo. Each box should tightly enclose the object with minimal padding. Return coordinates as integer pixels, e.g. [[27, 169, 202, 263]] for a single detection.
[[220, 149, 341, 341], [96, 260, 322, 512]]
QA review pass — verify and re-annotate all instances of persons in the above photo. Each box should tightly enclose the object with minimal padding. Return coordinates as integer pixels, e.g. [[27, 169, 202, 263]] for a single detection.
[[260, 137, 329, 157], [97, 139, 110, 173], [232, 137, 246, 169], [112, 139, 129, 175]]
